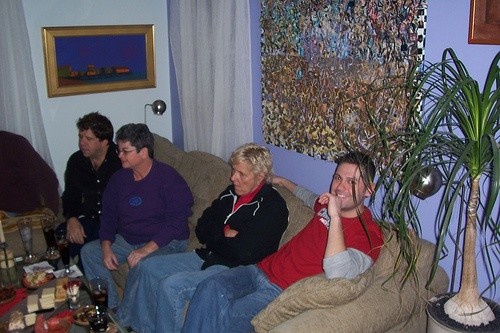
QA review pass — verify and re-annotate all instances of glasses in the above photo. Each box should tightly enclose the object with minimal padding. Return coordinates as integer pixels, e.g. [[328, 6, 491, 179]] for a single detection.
[[116, 147, 142, 156]]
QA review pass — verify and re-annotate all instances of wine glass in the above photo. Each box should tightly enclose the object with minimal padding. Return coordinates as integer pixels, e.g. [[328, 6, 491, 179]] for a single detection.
[[17, 215, 74, 277]]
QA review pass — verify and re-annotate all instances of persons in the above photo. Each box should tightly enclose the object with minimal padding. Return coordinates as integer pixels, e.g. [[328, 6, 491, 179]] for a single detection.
[[130, 143, 289, 333], [0, 131, 59, 217], [181, 153, 383, 333], [81, 122, 195, 333], [55, 112, 122, 275]]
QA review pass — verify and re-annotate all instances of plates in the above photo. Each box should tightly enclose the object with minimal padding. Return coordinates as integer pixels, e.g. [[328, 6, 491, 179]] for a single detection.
[[71, 312, 90, 326], [0, 274, 79, 333], [22, 270, 53, 289], [0, 287, 16, 305]]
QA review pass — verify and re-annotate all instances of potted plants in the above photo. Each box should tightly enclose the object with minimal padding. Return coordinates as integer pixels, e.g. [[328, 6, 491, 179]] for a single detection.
[[334, 47, 500, 333]]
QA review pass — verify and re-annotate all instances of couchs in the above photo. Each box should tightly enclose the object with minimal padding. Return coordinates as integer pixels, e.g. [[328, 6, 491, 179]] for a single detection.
[[113, 132, 449, 333]]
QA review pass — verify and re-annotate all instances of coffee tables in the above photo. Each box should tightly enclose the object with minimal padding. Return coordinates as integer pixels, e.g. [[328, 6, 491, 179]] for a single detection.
[[0, 252, 123, 333]]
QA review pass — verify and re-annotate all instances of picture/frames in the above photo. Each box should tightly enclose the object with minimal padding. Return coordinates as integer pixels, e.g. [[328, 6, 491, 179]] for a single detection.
[[41, 24, 157, 98]]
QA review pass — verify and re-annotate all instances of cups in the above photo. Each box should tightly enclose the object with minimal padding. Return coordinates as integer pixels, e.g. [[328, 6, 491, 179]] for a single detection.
[[66, 277, 81, 310], [0, 251, 18, 289], [83, 277, 109, 333]]
